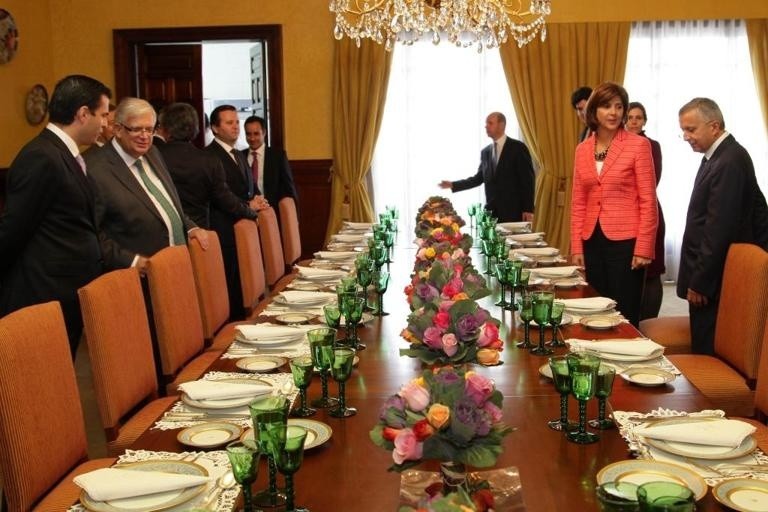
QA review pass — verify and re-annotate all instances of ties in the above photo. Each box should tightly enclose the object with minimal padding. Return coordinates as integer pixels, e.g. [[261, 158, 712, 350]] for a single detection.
[[231, 149, 248, 181], [134, 160, 186, 244], [251, 152, 261, 200], [494, 142, 497, 170]]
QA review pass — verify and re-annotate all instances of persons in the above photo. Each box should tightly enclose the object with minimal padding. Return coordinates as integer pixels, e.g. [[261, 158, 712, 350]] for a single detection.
[[754, 187, 767, 254], [570, 82, 659, 328], [4, 73, 302, 416], [572, 85, 596, 143], [624, 102, 667, 323], [436, 111, 536, 227], [674, 97, 757, 353]]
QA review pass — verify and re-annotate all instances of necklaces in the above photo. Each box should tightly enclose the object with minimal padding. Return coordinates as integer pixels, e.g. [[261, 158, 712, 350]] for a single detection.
[[594, 130, 612, 160]]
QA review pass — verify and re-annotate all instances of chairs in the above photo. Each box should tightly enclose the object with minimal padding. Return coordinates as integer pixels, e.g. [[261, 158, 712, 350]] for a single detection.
[[637, 243, 768, 458]]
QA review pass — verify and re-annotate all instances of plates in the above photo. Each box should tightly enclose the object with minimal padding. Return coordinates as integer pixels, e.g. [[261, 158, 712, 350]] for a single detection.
[[235, 325, 300, 345], [537, 359, 619, 382], [710, 476, 767, 512], [494, 220, 580, 289], [515, 312, 572, 326], [621, 367, 675, 386], [317, 313, 374, 326], [595, 458, 707, 503], [564, 305, 615, 314], [179, 377, 274, 411], [77, 460, 209, 512], [582, 338, 665, 362], [176, 420, 242, 447], [274, 312, 316, 324], [641, 414, 758, 461], [579, 316, 621, 331], [271, 221, 373, 308], [244, 417, 332, 451], [232, 355, 287, 373]]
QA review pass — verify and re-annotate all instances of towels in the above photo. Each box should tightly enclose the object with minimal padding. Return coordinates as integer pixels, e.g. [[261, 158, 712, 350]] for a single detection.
[[279, 222, 377, 304]]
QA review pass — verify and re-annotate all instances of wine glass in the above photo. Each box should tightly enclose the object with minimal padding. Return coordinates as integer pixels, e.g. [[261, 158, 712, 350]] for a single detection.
[[267, 424, 311, 512], [545, 354, 579, 431], [514, 296, 535, 351], [465, 203, 530, 312], [649, 496, 696, 512], [587, 364, 617, 428], [344, 298, 366, 350], [594, 481, 646, 511], [636, 480, 698, 509], [323, 304, 342, 348], [227, 439, 265, 512], [288, 356, 317, 416], [247, 395, 291, 507], [563, 350, 600, 443], [529, 291, 555, 355], [334, 283, 358, 344], [342, 202, 401, 315], [545, 302, 566, 347], [306, 327, 339, 408], [330, 346, 358, 418]]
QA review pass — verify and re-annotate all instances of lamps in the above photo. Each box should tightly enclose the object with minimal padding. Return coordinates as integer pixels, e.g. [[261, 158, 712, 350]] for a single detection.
[[329, 0, 551, 53]]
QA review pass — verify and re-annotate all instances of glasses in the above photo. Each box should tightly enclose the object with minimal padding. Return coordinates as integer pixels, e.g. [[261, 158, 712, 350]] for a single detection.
[[120, 122, 155, 135]]
